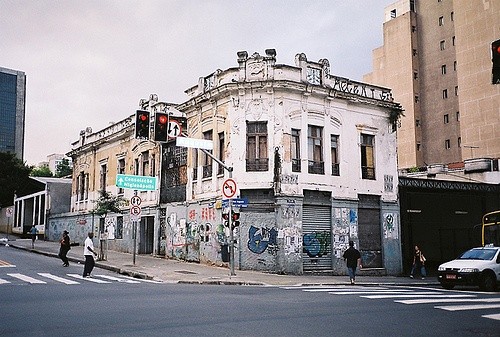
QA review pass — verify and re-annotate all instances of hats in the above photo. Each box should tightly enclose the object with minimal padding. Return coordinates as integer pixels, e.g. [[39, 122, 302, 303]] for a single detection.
[[349, 241, 354, 246]]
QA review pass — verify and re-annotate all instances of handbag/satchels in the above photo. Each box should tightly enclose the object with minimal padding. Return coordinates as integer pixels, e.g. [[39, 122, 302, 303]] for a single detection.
[[420, 251, 426, 262]]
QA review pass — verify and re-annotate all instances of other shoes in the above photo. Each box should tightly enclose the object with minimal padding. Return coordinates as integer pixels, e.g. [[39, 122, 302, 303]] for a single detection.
[[421, 277, 425, 279], [83, 275, 93, 277], [410, 275, 414, 279], [63, 263, 68, 266], [351, 279, 354, 284]]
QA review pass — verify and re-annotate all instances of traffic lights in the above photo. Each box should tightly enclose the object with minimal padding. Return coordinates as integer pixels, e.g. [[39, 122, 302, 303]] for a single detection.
[[135, 110, 150, 141], [153, 112, 169, 143], [231, 210, 240, 231], [222, 212, 229, 228]]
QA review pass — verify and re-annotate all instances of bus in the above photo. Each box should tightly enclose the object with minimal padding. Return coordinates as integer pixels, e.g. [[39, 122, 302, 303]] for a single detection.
[[471, 210, 500, 248]]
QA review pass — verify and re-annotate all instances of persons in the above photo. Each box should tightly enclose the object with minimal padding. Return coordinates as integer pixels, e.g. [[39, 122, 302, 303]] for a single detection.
[[58, 230, 71, 266], [410, 244, 426, 280], [341, 241, 361, 283], [30, 225, 38, 244], [83, 233, 98, 277]]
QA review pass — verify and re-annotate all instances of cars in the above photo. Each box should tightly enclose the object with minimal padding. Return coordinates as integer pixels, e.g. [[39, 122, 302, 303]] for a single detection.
[[436, 244, 500, 292]]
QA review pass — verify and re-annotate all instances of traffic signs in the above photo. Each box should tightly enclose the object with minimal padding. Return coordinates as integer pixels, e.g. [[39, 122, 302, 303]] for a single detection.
[[116, 174, 156, 191]]
